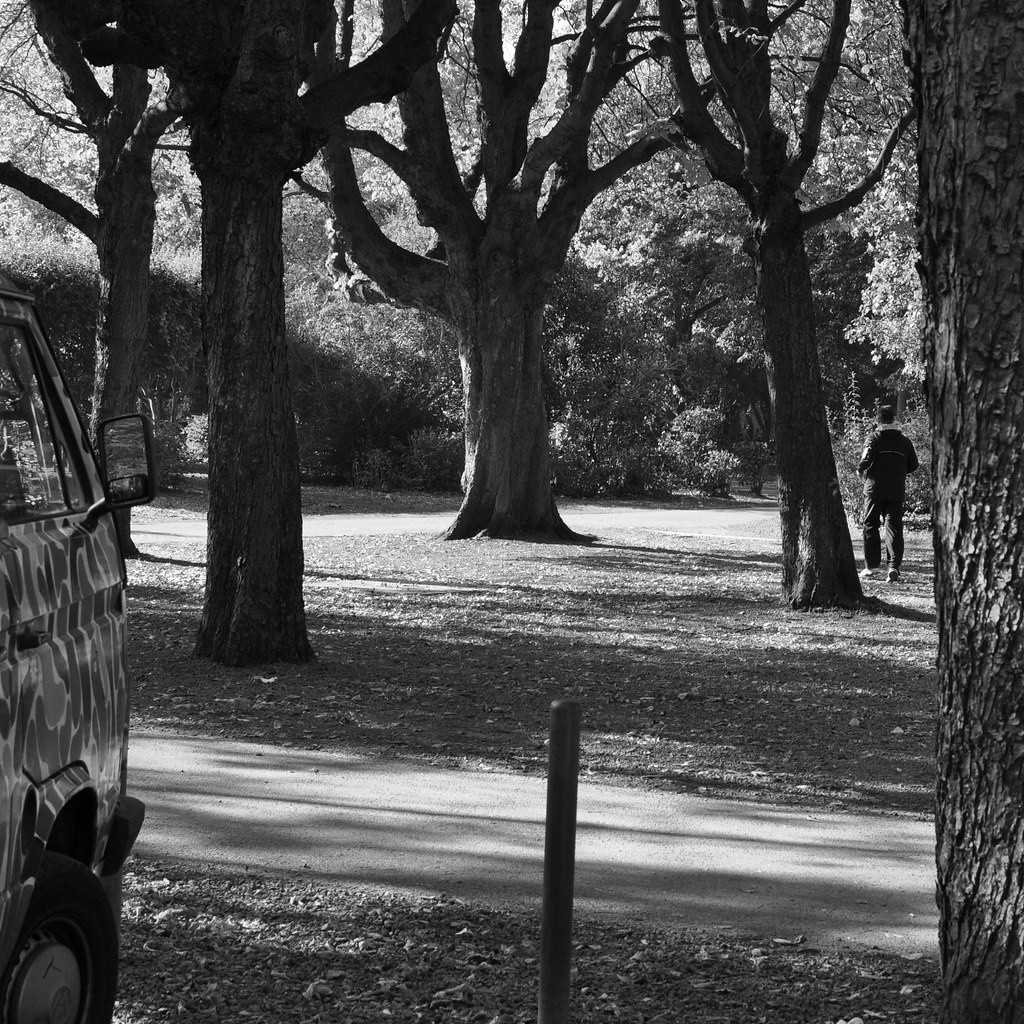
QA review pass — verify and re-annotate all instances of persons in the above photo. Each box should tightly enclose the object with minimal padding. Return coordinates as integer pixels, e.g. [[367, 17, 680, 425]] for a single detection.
[[857, 406, 919, 582]]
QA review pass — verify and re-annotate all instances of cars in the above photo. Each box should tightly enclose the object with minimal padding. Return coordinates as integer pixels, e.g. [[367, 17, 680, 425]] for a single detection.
[[0, 276, 156, 1024]]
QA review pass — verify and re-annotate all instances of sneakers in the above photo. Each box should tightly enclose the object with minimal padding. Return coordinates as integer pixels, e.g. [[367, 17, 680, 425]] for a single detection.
[[886, 568, 899, 583], [859, 567, 880, 576]]
